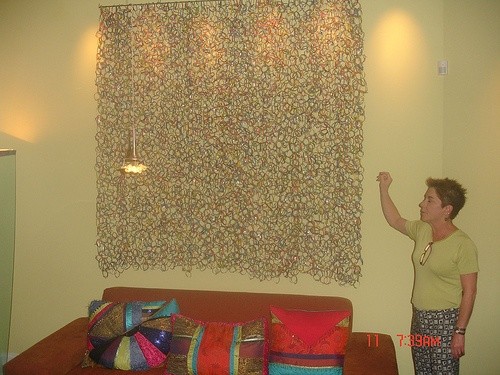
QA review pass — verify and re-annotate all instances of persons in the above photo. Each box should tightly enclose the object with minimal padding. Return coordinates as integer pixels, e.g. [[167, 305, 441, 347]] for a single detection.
[[376, 170, 481, 375]]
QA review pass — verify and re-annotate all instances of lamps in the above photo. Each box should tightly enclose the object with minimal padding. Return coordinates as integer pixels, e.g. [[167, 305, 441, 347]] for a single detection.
[[121, 0, 148, 173]]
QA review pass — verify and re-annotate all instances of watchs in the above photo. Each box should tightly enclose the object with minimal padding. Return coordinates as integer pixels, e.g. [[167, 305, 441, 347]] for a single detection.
[[453, 327, 466, 336]]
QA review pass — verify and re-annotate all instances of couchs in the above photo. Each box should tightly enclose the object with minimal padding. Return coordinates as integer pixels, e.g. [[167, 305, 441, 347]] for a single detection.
[[4, 287, 399, 375]]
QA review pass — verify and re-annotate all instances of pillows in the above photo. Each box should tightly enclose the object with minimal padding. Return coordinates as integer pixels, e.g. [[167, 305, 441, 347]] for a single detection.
[[82, 298, 178, 367], [168, 312, 269, 375], [266, 305, 351, 375]]
